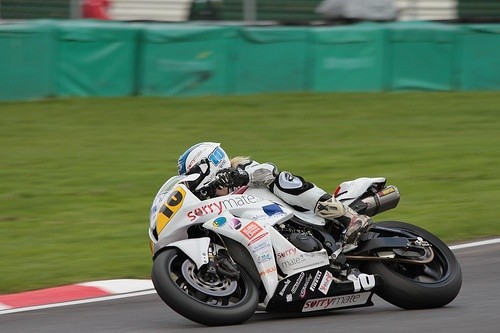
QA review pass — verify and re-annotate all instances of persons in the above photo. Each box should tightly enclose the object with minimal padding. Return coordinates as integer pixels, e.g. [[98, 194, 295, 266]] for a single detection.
[[177, 142, 373, 245]]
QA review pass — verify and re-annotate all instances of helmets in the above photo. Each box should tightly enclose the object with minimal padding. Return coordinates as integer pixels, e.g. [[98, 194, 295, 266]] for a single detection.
[[177, 141, 232, 198]]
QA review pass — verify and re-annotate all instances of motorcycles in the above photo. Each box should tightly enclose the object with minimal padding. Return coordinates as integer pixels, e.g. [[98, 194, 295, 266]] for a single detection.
[[148, 169, 463, 326]]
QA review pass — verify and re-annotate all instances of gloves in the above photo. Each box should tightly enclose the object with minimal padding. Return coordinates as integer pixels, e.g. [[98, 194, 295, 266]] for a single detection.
[[213, 168, 249, 188]]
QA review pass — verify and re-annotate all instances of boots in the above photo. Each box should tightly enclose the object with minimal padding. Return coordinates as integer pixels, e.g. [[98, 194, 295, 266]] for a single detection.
[[314, 194, 373, 242]]
